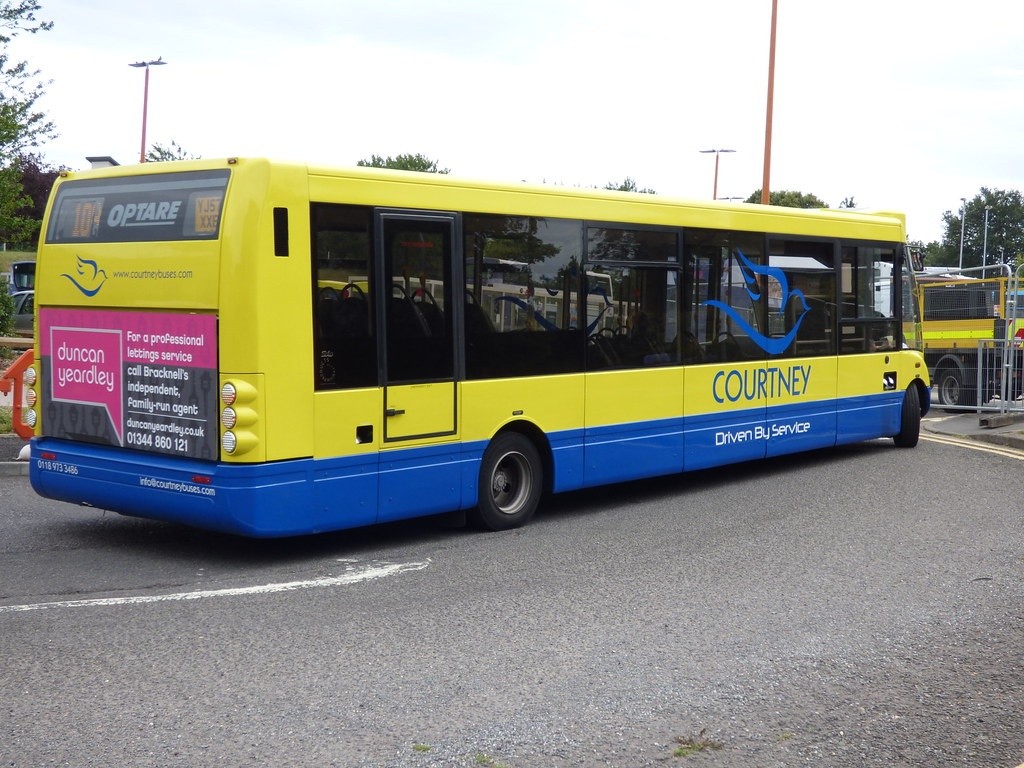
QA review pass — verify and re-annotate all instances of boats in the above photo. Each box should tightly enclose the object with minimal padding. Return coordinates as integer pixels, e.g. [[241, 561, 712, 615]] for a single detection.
[[4, 260, 36, 331]]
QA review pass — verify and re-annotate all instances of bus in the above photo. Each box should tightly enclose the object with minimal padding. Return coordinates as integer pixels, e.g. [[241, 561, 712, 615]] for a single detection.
[[28, 160, 932, 538], [464, 257, 614, 338]]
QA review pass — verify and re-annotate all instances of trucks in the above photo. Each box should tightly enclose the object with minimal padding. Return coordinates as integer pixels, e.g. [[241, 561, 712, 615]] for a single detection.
[[869, 262, 1024, 413]]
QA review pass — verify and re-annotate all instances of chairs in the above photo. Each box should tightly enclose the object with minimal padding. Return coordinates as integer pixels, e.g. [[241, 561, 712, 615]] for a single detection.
[[315, 282, 496, 337], [587, 326, 747, 368]]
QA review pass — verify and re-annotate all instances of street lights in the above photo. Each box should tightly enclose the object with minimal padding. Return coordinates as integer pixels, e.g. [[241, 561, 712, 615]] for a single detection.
[[699, 148, 737, 202], [983, 205, 992, 279], [128, 57, 169, 162], [958, 197, 967, 274]]
